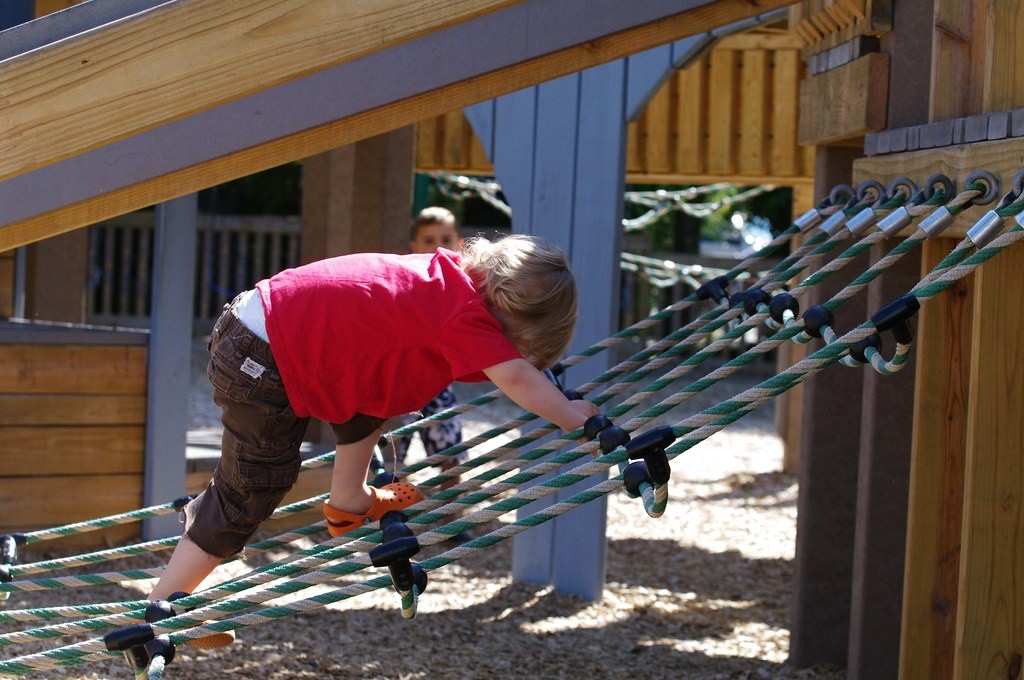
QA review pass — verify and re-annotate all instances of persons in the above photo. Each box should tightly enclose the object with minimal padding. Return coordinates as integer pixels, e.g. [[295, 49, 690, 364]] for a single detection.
[[377, 205, 475, 548], [121, 232, 579, 680]]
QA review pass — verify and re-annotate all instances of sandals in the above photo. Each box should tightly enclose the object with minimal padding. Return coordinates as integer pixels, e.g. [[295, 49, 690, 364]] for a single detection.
[[322, 482, 424, 536], [178, 620, 235, 649]]
